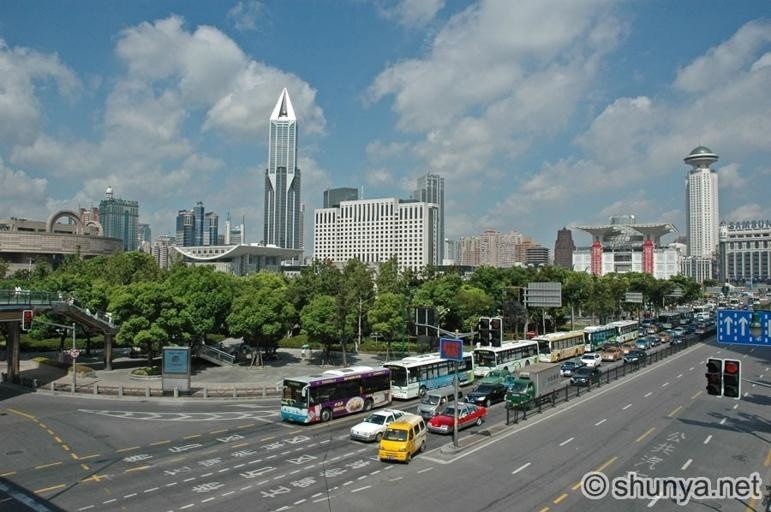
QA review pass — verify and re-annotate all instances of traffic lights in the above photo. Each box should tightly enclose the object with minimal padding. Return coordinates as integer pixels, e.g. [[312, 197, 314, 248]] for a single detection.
[[478, 316, 503, 347], [706, 357, 741, 400], [23, 311, 32, 330]]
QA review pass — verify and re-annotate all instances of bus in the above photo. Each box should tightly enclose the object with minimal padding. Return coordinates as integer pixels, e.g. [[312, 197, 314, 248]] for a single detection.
[[279, 305, 711, 426]]
